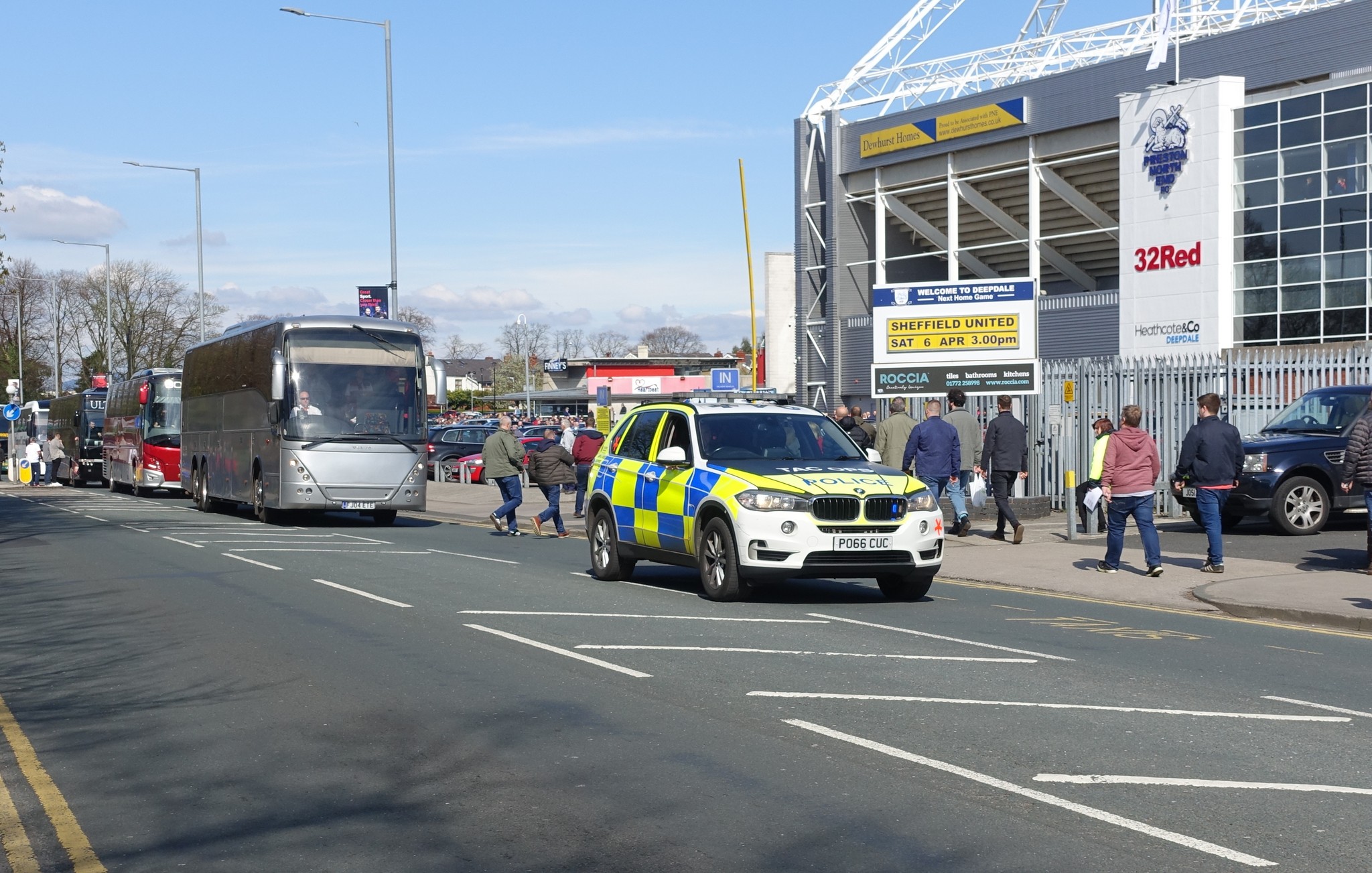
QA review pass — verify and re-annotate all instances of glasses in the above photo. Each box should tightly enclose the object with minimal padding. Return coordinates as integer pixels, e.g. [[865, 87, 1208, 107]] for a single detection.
[[946, 399, 950, 403], [300, 397, 309, 400], [1119, 416, 1128, 420]]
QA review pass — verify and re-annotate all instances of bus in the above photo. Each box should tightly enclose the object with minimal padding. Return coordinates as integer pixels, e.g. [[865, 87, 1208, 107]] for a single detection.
[[102, 367, 185, 497], [179, 313, 447, 528], [46, 385, 110, 489], [0, 400, 50, 476]]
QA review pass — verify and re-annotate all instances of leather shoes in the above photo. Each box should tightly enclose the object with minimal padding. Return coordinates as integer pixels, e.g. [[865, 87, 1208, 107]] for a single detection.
[[1097, 524, 1109, 532]]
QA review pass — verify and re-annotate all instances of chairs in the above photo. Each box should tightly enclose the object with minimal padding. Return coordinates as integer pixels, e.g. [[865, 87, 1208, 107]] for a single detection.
[[710, 418, 792, 459], [1333, 396, 1365, 426]]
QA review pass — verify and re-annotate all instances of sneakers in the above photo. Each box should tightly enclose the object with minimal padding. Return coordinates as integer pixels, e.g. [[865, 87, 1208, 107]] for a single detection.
[[507, 529, 524, 536], [989, 530, 1005, 541], [560, 488, 567, 493], [1097, 561, 1117, 573], [1200, 563, 1224, 573], [558, 531, 570, 538], [1202, 560, 1212, 566], [1145, 564, 1164, 577], [530, 517, 542, 536], [565, 489, 575, 495], [1013, 524, 1024, 544], [958, 517, 971, 537], [490, 513, 503, 531], [948, 521, 961, 534]]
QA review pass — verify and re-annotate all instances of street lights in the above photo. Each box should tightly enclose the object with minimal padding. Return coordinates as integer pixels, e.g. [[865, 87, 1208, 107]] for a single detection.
[[52, 238, 113, 386], [11, 276, 59, 399], [492, 361, 496, 418], [530, 367, 537, 417], [276, 6, 398, 320], [480, 367, 485, 415], [122, 161, 204, 343]]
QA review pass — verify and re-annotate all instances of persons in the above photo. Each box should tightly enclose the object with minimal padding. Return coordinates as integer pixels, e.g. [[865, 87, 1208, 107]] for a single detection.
[[0, 442, 5, 481], [1173, 393, 1244, 573], [290, 391, 323, 418], [42, 433, 65, 487], [481, 415, 527, 536], [25, 437, 43, 486], [558, 419, 575, 495], [1341, 393, 1372, 575], [154, 407, 176, 428], [619, 403, 626, 414], [980, 395, 1028, 544], [428, 410, 620, 429], [573, 417, 605, 517], [528, 428, 578, 538], [851, 406, 876, 444], [824, 410, 877, 421], [86, 420, 102, 440], [822, 406, 872, 461], [1076, 405, 1163, 576], [363, 306, 386, 319], [807, 421, 824, 455], [873, 389, 983, 537]]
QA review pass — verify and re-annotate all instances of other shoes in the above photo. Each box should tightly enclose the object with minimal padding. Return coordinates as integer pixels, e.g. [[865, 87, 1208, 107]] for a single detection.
[[52, 482, 62, 486], [35, 484, 41, 487], [45, 484, 52, 487], [573, 513, 582, 518]]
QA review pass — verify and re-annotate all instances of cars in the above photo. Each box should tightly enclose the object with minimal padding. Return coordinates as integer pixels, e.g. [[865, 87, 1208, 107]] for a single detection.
[[1167, 385, 1372, 536], [416, 410, 598, 486]]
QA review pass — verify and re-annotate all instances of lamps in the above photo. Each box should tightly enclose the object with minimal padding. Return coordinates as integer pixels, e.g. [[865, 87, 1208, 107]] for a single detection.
[[620, 406, 626, 414], [608, 377, 613, 381], [681, 377, 685, 380]]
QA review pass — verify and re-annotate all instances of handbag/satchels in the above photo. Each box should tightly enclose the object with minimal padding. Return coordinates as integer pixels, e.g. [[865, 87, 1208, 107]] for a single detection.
[[970, 472, 987, 507]]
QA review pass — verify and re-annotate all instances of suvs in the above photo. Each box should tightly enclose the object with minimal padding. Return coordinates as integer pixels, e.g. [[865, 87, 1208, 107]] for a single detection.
[[583, 368, 945, 603]]
